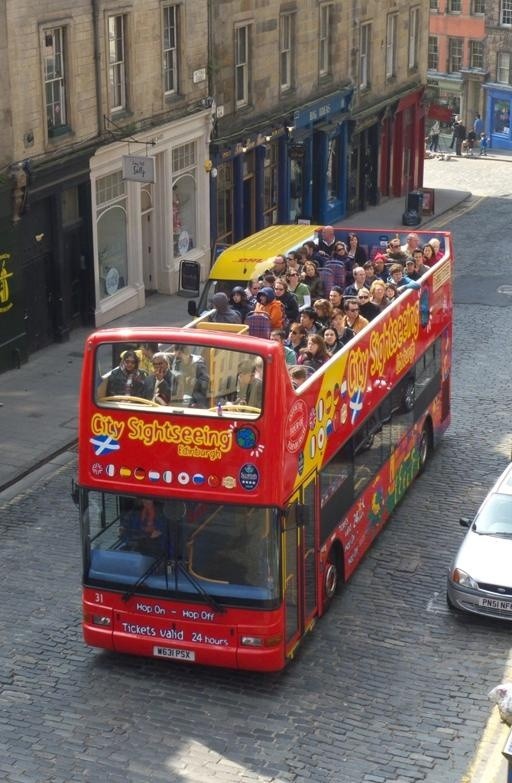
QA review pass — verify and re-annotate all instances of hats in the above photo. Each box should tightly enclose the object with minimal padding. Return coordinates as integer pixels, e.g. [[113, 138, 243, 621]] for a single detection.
[[374, 254, 384, 261], [405, 256, 416, 264]]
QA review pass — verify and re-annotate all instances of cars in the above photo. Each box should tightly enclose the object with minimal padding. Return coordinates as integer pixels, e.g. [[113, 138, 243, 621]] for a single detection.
[[446, 462, 512, 622]]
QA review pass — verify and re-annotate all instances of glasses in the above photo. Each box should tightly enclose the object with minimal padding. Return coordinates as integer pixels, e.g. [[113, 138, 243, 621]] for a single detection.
[[287, 258, 293, 261], [394, 244, 401, 248], [338, 247, 344, 250], [290, 330, 297, 335], [126, 360, 137, 364], [352, 308, 360, 312], [359, 296, 369, 299], [275, 288, 284, 291], [290, 273, 300, 276]]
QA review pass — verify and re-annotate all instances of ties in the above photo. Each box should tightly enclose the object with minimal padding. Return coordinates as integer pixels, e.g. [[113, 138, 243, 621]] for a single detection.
[[143, 512, 151, 528]]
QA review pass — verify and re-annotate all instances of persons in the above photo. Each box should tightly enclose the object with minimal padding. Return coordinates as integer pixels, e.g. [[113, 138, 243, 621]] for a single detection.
[[173, 185, 183, 235], [473, 113, 484, 148], [448, 114, 460, 147], [453, 119, 466, 155], [107, 343, 207, 408], [429, 119, 440, 151], [212, 225, 443, 389], [291, 157, 306, 217], [135, 519, 160, 553], [476, 131, 491, 156], [217, 357, 263, 413], [465, 126, 477, 155]]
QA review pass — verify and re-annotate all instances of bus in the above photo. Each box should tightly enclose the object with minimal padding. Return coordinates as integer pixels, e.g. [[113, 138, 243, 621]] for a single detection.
[[74, 224, 454, 674]]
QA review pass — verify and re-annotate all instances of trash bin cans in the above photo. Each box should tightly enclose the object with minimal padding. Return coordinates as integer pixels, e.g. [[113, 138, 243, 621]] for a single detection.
[[407, 192, 423, 224]]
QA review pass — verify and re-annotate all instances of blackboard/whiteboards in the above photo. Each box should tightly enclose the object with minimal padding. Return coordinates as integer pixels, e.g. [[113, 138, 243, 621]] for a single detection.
[[212, 243, 231, 268], [182, 262, 200, 290]]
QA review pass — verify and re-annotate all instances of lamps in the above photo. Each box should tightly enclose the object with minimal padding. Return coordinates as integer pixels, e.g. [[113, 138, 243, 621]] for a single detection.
[[263, 128, 273, 143], [286, 121, 296, 133], [240, 140, 249, 154], [205, 159, 213, 171]]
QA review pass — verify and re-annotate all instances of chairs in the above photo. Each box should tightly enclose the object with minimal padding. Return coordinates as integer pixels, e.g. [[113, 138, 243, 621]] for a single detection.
[[316, 270, 335, 298], [247, 312, 273, 340], [360, 245, 369, 260], [370, 246, 386, 260], [326, 261, 346, 287]]
